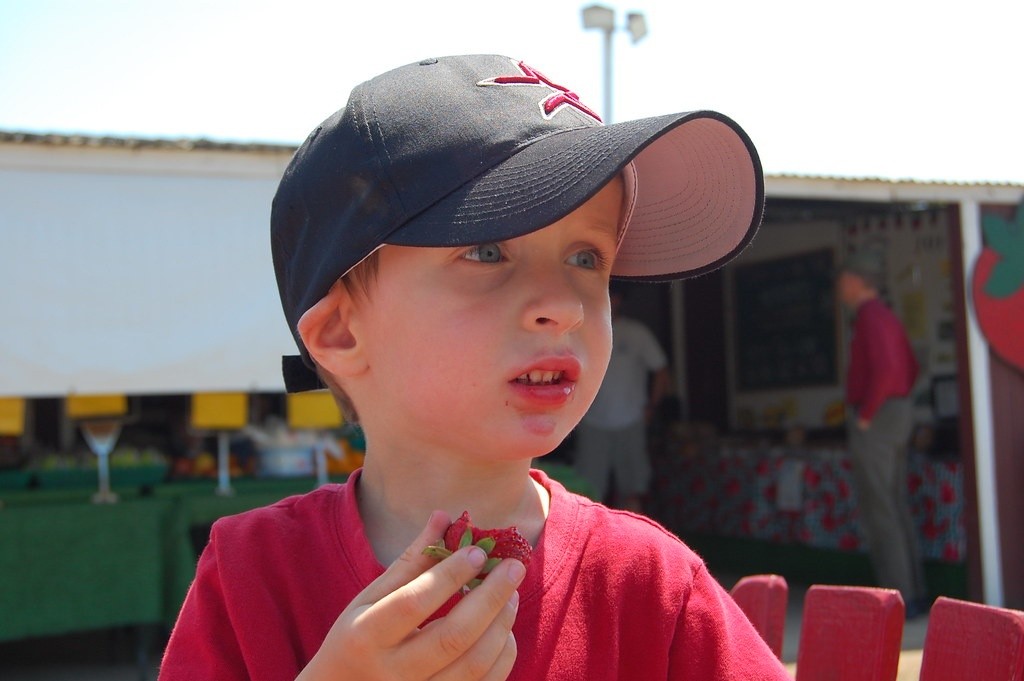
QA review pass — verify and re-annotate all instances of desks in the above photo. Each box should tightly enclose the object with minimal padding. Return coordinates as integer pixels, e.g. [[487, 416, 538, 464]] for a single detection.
[[0, 463, 346, 681], [648, 437, 966, 616]]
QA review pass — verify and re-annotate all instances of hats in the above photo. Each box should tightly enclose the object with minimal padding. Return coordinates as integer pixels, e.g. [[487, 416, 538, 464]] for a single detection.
[[269, 54, 765, 371], [844, 246, 905, 293]]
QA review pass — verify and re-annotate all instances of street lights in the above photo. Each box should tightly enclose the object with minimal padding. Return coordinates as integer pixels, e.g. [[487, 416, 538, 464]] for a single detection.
[[582, 1, 650, 126]]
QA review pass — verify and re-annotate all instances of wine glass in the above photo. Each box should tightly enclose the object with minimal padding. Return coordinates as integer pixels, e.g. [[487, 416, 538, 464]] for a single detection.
[[79, 420, 122, 503]]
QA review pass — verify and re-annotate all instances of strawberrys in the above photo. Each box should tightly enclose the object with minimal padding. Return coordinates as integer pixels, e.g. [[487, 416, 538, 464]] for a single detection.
[[425, 509, 536, 596]]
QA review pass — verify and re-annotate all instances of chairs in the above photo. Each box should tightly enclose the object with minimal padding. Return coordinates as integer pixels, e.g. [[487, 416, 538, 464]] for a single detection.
[[796, 585, 905, 681], [919, 596, 1024, 681], [728, 574, 788, 660]]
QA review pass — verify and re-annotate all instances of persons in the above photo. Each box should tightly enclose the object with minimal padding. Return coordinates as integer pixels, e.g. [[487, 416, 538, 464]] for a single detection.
[[836, 261, 930, 620], [577, 294, 671, 513], [158, 52, 795, 681]]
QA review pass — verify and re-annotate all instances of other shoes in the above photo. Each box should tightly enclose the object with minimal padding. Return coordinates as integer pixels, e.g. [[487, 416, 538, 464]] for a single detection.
[[904, 595, 930, 620]]
